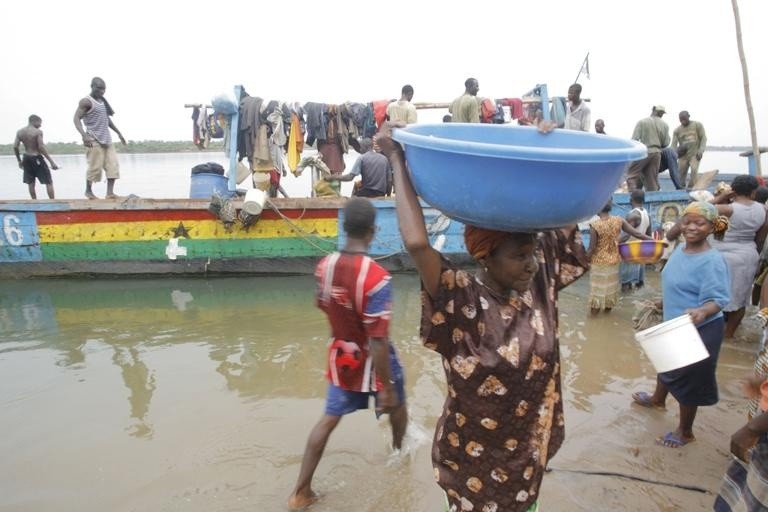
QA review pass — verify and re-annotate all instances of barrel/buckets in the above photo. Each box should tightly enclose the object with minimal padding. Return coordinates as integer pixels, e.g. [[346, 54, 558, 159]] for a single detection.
[[634, 312, 711, 374]]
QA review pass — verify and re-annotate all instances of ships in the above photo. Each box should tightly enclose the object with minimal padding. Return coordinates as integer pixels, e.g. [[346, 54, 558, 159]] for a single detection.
[[0, 147, 768, 280]]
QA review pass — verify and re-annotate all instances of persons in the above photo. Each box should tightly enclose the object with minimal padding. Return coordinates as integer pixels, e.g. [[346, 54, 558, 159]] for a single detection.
[[688, 189, 714, 202], [714, 182, 731, 204], [759, 233, 768, 310], [377, 120, 590, 512], [564, 84, 592, 131], [595, 119, 606, 134], [586, 197, 669, 314], [291, 199, 407, 511], [617, 190, 652, 292], [637, 146, 688, 190], [13, 116, 58, 199], [752, 187, 768, 306], [326, 138, 392, 198], [706, 175, 768, 340], [747, 342, 767, 420], [73, 78, 127, 200], [448, 78, 480, 123], [626, 104, 670, 192], [672, 110, 707, 191], [386, 85, 417, 124], [714, 411, 768, 512], [632, 202, 732, 448]]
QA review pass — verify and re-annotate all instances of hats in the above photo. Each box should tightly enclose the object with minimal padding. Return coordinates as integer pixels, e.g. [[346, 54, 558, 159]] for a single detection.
[[681, 200, 729, 242], [462, 223, 508, 261], [651, 104, 667, 114]]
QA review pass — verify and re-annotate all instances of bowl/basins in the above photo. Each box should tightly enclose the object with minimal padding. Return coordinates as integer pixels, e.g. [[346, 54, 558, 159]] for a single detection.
[[389, 121, 648, 233], [618, 240, 669, 265]]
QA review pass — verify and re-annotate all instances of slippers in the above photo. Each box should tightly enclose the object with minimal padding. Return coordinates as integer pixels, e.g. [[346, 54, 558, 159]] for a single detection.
[[632, 391, 666, 412], [657, 432, 697, 448]]
